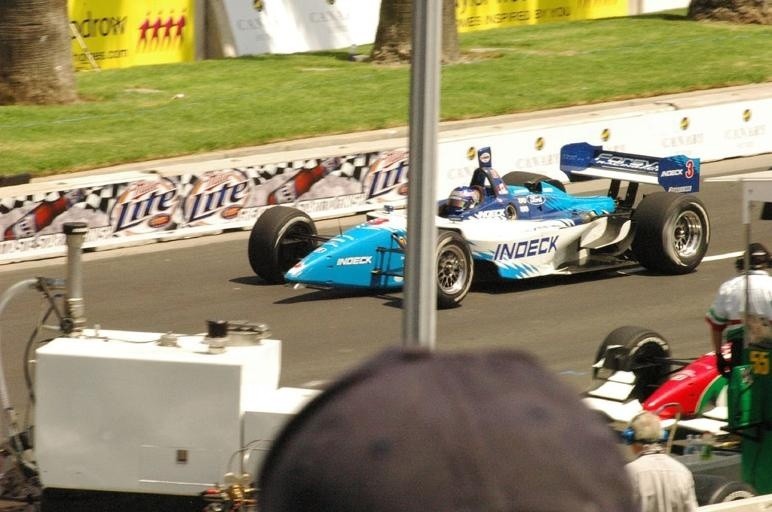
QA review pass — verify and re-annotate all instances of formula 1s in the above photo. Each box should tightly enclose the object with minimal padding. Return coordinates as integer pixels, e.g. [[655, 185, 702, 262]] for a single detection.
[[247, 141, 710, 306], [577, 324, 759, 506]]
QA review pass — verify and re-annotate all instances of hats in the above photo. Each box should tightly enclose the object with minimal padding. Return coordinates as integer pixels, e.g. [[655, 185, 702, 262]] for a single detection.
[[629, 411, 663, 440], [749, 243, 768, 264]]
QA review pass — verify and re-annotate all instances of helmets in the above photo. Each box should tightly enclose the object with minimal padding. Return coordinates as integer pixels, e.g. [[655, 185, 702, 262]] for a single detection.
[[445, 186, 481, 216]]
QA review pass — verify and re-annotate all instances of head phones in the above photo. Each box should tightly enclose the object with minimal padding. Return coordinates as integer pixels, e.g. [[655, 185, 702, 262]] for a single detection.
[[735, 247, 772, 270], [622, 414, 670, 444]]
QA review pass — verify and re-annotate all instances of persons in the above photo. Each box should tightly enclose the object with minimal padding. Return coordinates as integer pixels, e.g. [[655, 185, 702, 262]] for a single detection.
[[440, 184, 482, 217], [705, 239, 770, 494], [618, 411, 705, 511]]
